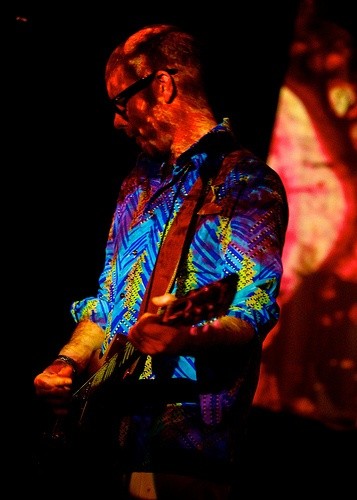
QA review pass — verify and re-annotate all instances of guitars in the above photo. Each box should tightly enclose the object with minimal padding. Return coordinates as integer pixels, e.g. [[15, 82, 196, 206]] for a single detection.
[[52, 272, 240, 441]]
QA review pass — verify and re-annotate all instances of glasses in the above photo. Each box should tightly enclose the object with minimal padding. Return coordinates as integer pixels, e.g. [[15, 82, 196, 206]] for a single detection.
[[112, 68, 179, 114]]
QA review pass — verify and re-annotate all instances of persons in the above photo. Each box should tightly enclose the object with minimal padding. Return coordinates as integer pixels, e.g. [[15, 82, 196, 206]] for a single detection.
[[33, 21, 288, 500]]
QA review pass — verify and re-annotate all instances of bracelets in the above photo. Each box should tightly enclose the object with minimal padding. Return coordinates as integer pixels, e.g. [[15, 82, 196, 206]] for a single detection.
[[51, 354, 80, 378]]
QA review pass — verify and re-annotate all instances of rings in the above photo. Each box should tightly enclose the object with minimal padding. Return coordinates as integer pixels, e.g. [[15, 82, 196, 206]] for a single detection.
[[135, 337, 144, 344]]
